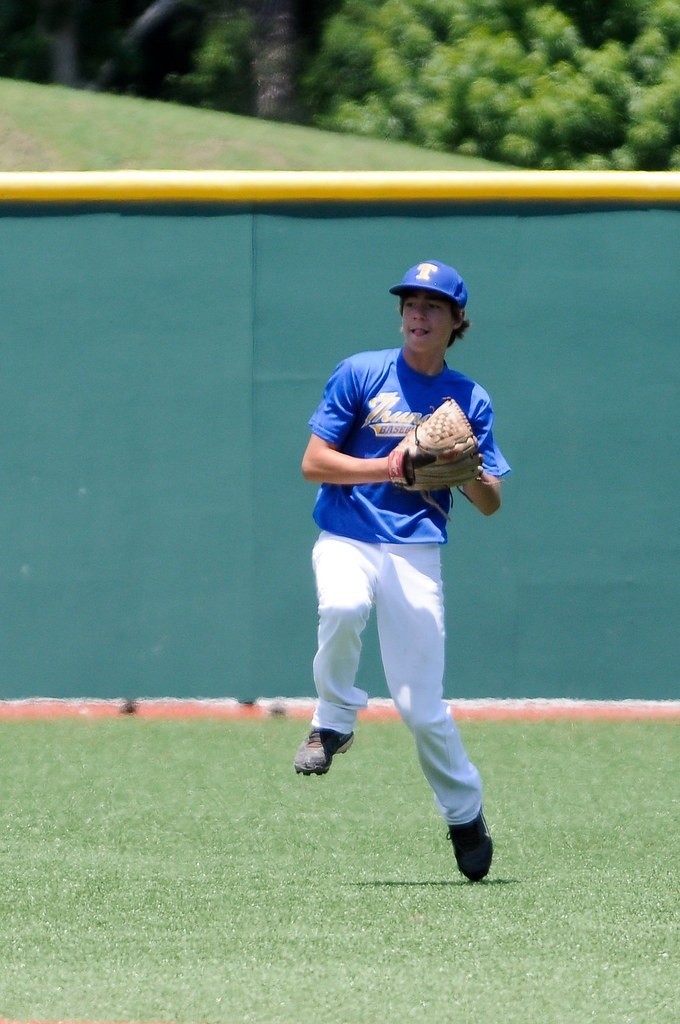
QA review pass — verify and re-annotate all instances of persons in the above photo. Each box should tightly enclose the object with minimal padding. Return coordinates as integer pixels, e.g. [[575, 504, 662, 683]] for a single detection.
[[295, 260, 511, 881]]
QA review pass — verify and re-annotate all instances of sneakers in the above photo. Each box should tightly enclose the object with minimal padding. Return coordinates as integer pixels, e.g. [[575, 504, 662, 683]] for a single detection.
[[294, 727, 355, 776], [445, 807, 493, 882]]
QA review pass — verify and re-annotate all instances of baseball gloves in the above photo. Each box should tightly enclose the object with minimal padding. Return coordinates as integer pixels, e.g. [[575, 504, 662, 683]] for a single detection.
[[384, 393, 486, 497]]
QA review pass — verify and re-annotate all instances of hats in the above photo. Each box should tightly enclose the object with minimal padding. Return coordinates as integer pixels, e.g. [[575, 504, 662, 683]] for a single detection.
[[388, 260, 468, 309]]
